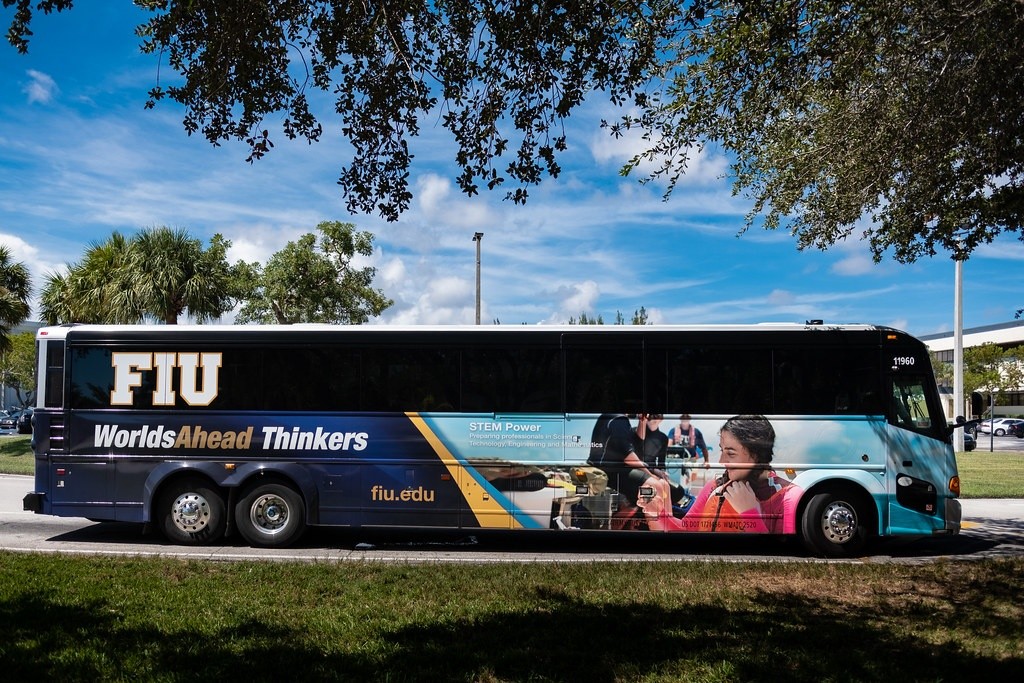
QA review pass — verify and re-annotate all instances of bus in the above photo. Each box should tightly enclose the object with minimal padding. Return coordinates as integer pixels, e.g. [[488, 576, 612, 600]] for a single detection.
[[22, 319, 988, 560]]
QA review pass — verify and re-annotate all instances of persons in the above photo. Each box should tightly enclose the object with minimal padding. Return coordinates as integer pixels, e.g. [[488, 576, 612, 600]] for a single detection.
[[586, 415, 710, 533], [636, 416, 803, 535]]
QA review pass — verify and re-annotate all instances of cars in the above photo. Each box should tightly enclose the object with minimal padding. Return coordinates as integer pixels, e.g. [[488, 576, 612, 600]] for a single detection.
[[964, 418, 986, 434], [946, 423, 977, 452], [980, 417, 1024, 437], [1007, 421, 1024, 439], [0, 404, 34, 434]]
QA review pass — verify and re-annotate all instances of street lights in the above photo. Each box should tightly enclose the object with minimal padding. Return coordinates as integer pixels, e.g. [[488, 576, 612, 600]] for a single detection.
[[471, 232, 485, 327]]
